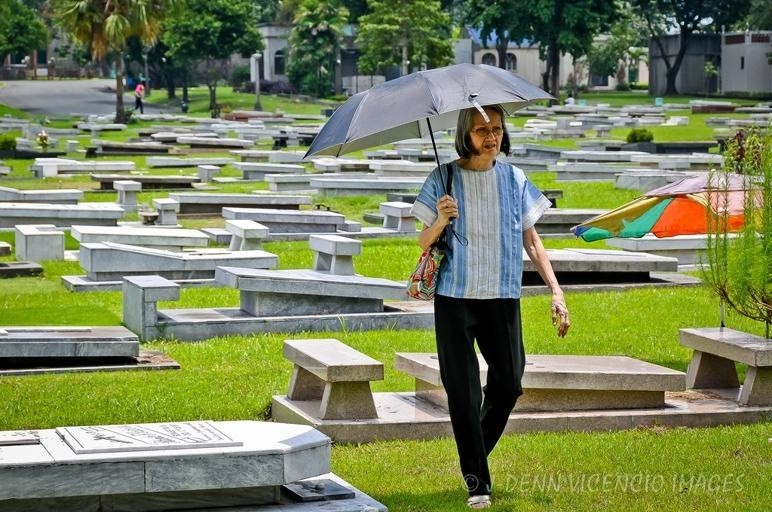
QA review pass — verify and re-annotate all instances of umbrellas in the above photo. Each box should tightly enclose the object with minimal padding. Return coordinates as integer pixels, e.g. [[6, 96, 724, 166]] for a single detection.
[[303, 55, 557, 224], [569, 165, 771, 329]]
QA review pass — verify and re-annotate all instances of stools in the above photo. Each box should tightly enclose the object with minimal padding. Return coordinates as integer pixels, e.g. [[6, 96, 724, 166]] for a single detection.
[[0, 102, 772, 421]]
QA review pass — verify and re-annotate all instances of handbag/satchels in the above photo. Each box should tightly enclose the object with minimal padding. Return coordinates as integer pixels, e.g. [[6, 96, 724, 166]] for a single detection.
[[406, 246, 444, 302]]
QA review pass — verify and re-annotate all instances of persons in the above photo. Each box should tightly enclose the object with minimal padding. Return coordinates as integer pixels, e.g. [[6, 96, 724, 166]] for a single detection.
[[129, 77, 145, 114], [563, 94, 575, 106], [410, 101, 571, 508]]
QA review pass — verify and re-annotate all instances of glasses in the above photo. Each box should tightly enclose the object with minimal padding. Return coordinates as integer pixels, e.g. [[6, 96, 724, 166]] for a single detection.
[[470, 126, 505, 136]]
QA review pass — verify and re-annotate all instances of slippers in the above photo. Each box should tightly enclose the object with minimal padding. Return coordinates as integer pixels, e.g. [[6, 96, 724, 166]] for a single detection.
[[467, 495, 491, 510]]
